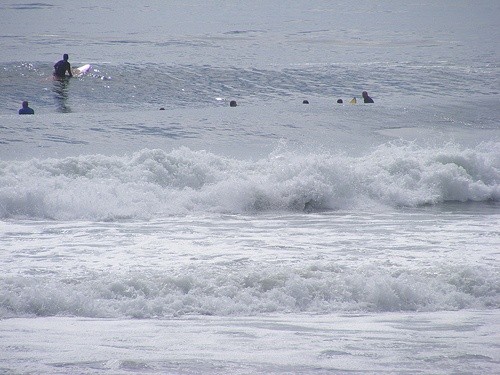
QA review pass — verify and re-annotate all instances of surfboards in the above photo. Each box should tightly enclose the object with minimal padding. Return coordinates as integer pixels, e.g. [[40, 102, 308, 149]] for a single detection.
[[72, 64, 92, 78]]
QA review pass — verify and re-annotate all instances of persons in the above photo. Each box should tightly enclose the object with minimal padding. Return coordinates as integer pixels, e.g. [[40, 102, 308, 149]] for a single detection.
[[18, 101, 34, 115], [53, 53, 73, 78], [362, 91, 374, 103]]
